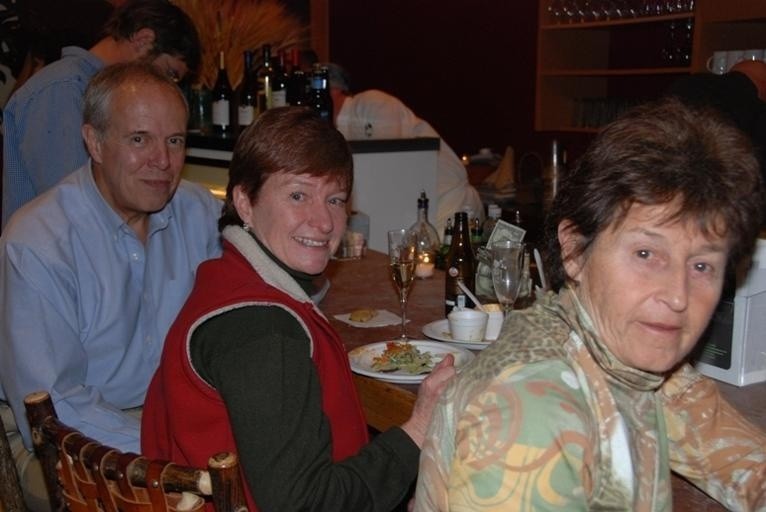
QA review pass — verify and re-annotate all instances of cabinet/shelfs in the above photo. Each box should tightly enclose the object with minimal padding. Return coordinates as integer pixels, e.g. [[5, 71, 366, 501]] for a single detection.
[[535, 0, 765, 144]]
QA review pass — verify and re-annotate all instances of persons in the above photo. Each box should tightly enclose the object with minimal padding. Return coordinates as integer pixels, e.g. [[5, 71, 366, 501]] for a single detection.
[[413, 96, 765, 511], [0, 1, 202, 234], [139, 103, 459, 509], [0, 60, 227, 457], [312, 61, 487, 262]]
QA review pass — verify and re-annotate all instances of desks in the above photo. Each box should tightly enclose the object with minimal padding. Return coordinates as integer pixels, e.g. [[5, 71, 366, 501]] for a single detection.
[[309, 243, 447, 441], [669, 369, 765, 510]]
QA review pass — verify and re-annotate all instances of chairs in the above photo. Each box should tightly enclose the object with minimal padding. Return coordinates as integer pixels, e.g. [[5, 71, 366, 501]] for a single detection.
[[21, 388, 251, 512]]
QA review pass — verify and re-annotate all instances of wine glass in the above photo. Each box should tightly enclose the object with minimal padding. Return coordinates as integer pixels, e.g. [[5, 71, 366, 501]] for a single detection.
[[543, 3, 693, 23], [386, 228, 420, 340]]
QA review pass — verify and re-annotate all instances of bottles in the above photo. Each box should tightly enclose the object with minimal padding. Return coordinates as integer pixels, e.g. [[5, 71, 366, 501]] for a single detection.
[[482, 204, 504, 247], [405, 188, 441, 280], [190, 40, 333, 155], [440, 218, 454, 268], [657, 19, 693, 67], [445, 210, 476, 316], [471, 219, 483, 250], [491, 240, 524, 316], [512, 207, 533, 310]]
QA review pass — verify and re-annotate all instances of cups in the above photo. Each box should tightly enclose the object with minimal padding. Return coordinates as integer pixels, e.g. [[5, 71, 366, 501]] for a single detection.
[[705, 49, 765, 75], [478, 302, 504, 340], [568, 96, 652, 130], [413, 249, 436, 279], [448, 310, 489, 340]]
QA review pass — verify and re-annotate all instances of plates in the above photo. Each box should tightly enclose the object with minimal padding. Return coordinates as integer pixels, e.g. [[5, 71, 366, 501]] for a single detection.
[[345, 339, 480, 384], [421, 318, 496, 348]]
